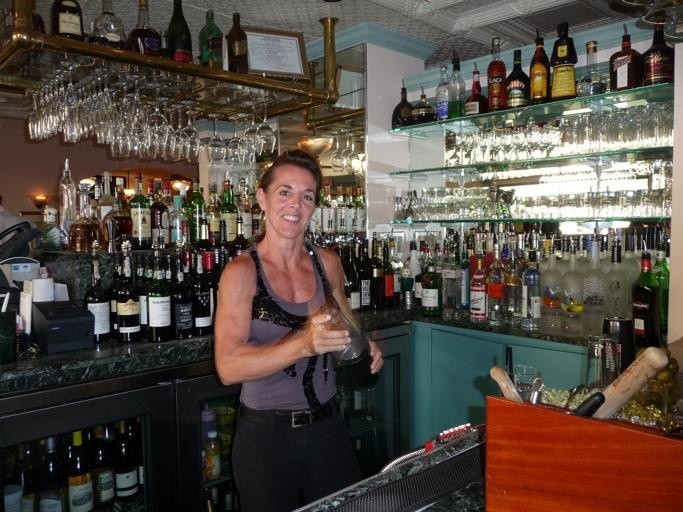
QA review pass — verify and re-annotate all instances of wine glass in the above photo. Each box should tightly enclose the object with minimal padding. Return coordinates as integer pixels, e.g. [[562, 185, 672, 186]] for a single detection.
[[23, 58, 278, 165], [328, 130, 366, 175]]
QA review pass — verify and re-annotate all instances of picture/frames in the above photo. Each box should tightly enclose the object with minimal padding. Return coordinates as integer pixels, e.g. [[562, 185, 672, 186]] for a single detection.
[[244, 25, 311, 80]]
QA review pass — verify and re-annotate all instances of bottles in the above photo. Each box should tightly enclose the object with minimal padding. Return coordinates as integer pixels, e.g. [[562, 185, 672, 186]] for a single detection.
[[314, 227, 670, 344], [1, 1, 247, 70], [392, 13, 676, 130], [198, 402, 238, 512], [50, 158, 366, 349], [0, 413, 149, 510], [317, 305, 380, 394]]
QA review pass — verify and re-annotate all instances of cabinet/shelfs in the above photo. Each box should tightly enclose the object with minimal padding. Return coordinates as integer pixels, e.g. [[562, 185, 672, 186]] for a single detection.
[[410, 322, 588, 448], [388, 82, 673, 226], [0, 325, 412, 512]]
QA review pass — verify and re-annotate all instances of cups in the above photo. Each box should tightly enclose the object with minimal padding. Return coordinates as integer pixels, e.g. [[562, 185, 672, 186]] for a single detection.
[[586, 334, 620, 390], [501, 362, 541, 404], [391, 96, 677, 224], [621, 382, 669, 418]]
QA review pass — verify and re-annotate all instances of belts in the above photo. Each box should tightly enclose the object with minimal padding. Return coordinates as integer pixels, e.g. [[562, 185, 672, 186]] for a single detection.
[[238, 397, 341, 430]]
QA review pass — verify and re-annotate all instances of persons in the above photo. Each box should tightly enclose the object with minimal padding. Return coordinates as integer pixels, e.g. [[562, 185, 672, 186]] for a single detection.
[[213, 149, 384, 512]]
